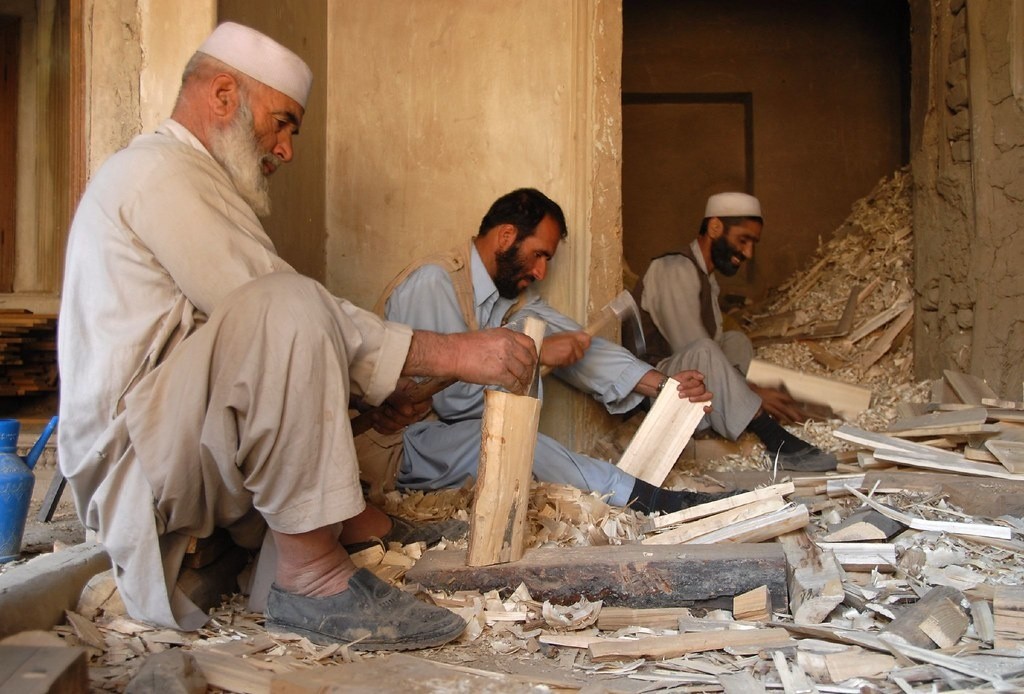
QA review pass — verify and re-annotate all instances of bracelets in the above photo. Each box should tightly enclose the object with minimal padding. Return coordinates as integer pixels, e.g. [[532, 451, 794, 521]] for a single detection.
[[657, 375, 669, 396]]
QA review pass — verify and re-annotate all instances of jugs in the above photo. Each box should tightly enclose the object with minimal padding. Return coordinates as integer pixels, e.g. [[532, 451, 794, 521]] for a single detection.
[[0, 415, 60, 562]]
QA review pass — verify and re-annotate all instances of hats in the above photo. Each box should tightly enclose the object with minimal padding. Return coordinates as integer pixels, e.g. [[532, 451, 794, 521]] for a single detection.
[[704, 192, 763, 217], [197, 21, 314, 115]]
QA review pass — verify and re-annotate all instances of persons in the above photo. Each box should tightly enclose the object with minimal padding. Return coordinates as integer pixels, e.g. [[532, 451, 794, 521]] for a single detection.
[[622, 192, 838, 472], [346, 187, 748, 517], [58, 21, 539, 652]]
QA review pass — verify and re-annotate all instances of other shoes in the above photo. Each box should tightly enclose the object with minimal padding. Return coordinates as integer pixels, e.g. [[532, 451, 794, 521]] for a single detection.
[[769, 443, 838, 471], [265, 569, 466, 652], [344, 513, 442, 555], [670, 488, 750, 516]]
